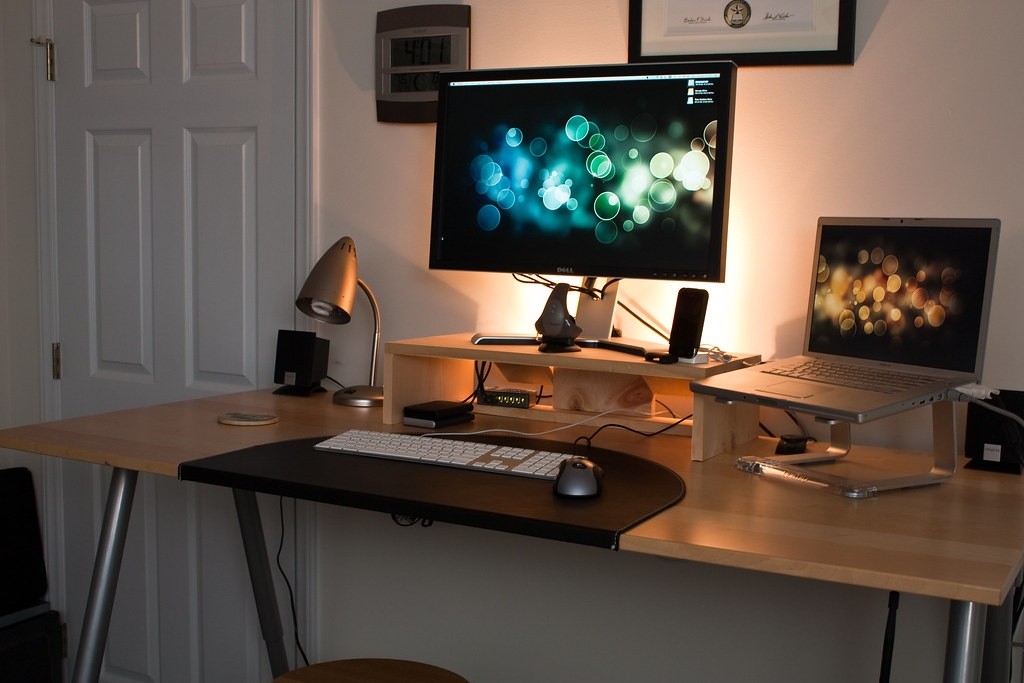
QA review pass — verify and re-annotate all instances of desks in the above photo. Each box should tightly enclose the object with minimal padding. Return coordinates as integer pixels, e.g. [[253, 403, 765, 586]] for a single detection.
[[0, 385, 1024, 683]]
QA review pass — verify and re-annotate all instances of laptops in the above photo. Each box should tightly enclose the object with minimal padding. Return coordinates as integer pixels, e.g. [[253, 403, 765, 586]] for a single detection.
[[690, 216, 1001, 424]]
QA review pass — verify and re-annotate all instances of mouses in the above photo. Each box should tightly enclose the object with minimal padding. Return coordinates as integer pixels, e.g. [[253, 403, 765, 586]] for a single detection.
[[774, 434, 817, 456], [551, 458, 604, 502]]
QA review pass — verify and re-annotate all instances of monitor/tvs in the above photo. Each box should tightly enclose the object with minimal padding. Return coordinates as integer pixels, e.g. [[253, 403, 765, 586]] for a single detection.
[[428, 61, 738, 357]]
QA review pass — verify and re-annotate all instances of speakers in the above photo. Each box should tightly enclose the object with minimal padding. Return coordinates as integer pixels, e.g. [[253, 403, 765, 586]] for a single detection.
[[272, 329, 330, 397], [962, 390, 1024, 475]]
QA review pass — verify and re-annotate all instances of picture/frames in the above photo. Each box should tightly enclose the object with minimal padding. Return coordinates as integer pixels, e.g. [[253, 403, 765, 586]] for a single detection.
[[628, 0, 856, 67]]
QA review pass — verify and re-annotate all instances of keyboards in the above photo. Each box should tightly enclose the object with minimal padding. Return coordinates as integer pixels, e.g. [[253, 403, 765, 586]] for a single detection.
[[313, 429, 587, 480]]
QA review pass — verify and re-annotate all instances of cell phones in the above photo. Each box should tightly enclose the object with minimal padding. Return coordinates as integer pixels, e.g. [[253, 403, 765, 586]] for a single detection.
[[668, 287, 710, 359]]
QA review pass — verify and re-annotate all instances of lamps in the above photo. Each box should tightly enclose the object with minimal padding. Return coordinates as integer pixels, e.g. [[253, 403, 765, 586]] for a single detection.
[[293, 235, 383, 408]]
